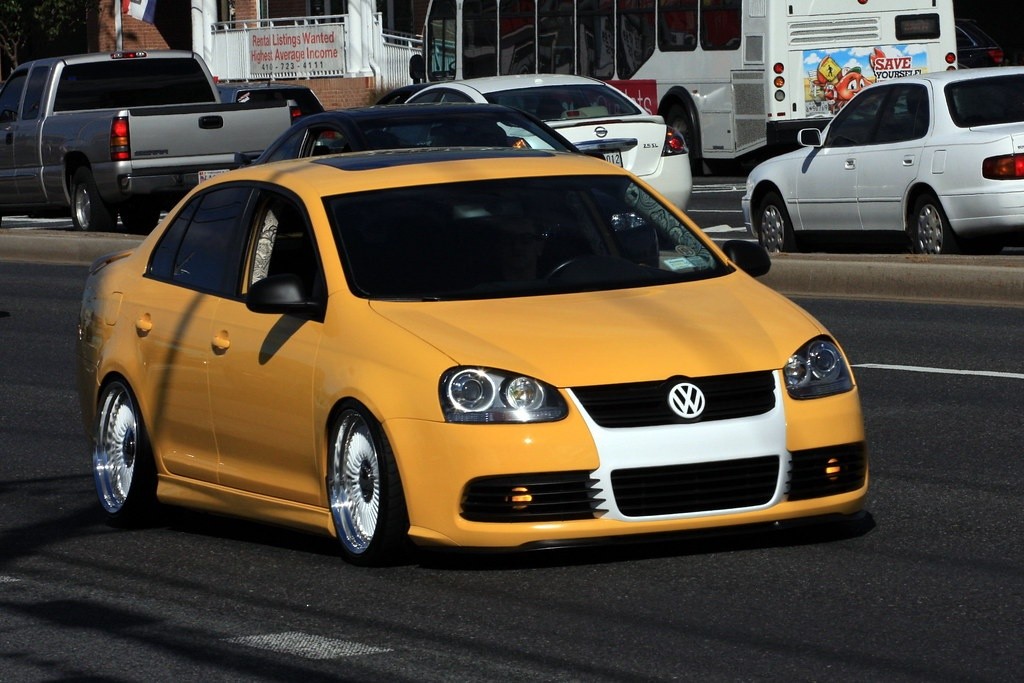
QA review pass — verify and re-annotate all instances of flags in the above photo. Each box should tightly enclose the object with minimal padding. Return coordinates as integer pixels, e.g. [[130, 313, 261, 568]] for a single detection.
[[123, 0, 156, 25]]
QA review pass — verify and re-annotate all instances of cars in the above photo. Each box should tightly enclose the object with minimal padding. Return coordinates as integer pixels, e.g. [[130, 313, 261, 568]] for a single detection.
[[741, 66, 1024, 253], [372, 74, 693, 220], [216, 81, 326, 126], [77, 143, 873, 565], [954, 16, 1008, 68]]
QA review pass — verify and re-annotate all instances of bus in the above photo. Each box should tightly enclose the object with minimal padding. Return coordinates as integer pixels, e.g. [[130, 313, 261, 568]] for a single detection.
[[408, 0, 958, 170]]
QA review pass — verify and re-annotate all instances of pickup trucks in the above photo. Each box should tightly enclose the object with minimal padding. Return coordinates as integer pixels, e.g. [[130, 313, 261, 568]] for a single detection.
[[0, 50, 299, 227]]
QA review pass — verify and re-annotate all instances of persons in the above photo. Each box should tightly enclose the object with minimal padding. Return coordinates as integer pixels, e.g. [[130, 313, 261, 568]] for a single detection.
[[485, 216, 579, 292]]
